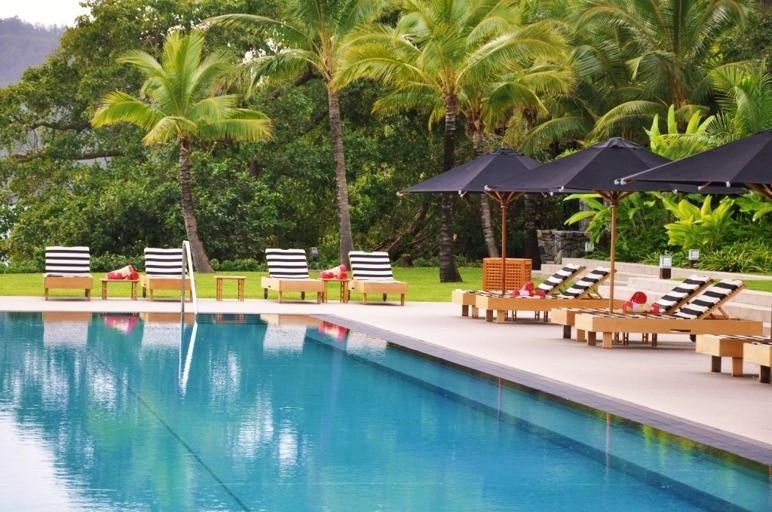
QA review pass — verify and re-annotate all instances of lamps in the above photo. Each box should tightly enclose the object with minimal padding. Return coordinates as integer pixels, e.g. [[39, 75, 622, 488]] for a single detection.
[[658, 255, 672, 280], [583, 241, 595, 259], [688, 249, 700, 268]]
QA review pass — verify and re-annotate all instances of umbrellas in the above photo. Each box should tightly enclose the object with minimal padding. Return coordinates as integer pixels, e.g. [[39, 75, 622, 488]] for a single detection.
[[401, 149, 597, 296], [614, 129, 772, 200], [484, 137, 750, 315]]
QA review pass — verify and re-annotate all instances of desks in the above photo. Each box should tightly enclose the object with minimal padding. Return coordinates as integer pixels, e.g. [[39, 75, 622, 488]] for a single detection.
[[216, 276, 246, 300], [99, 277, 140, 300], [315, 275, 351, 302]]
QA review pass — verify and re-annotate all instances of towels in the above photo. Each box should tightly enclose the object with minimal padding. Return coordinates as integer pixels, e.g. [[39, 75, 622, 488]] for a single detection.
[[129, 271, 139, 280], [336, 271, 349, 280], [320, 263, 348, 280], [108, 262, 137, 279]]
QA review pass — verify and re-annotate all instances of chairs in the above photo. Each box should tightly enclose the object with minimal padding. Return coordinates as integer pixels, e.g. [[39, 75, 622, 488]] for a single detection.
[[551, 275, 763, 349], [43, 243, 94, 300], [452, 264, 627, 323], [695, 334, 772, 376], [140, 247, 195, 302], [346, 249, 408, 303], [743, 342, 772, 383], [258, 247, 324, 305]]
